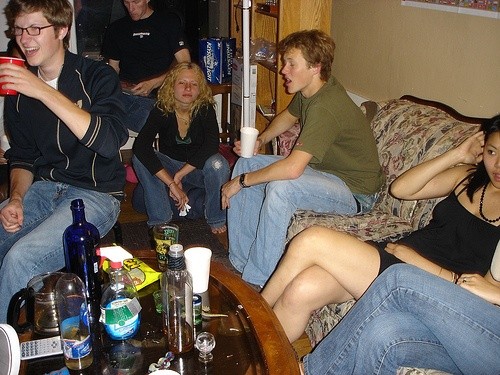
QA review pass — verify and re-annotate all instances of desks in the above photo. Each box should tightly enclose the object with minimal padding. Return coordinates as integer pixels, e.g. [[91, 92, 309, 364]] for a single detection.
[[6, 248, 299, 375]]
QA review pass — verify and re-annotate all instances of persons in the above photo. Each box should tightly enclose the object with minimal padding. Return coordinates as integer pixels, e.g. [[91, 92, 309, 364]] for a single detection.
[[0, 0, 230, 324], [222, 29, 380, 305], [260, 114, 500, 375]]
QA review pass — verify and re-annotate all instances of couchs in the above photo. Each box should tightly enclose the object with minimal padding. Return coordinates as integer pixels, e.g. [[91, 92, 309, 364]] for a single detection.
[[274, 93, 500, 375]]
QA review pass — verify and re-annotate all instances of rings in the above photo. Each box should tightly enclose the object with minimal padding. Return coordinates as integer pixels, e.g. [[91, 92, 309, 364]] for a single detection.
[[464, 278, 467, 282]]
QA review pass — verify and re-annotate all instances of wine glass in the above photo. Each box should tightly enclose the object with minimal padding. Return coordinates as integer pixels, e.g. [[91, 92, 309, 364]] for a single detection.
[[196, 333, 215, 362]]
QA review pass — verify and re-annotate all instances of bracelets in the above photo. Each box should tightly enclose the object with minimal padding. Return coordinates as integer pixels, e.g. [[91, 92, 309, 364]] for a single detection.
[[450, 272, 459, 282], [239, 174, 251, 188], [168, 182, 175, 186], [437, 267, 444, 277]]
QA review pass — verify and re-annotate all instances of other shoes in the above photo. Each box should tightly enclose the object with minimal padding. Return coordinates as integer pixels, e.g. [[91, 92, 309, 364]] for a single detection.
[[212, 224, 230, 250]]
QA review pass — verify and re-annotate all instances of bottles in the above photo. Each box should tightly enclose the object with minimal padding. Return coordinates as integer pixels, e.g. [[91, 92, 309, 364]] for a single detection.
[[63, 199, 102, 306], [165, 244, 194, 354], [101, 261, 141, 342], [55, 273, 95, 370]]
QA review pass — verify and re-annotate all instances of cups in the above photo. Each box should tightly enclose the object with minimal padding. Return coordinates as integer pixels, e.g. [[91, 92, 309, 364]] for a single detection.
[[241, 127, 260, 159], [154, 291, 164, 313], [0, 56, 26, 96], [193, 295, 203, 325], [185, 247, 214, 294], [153, 222, 179, 271]]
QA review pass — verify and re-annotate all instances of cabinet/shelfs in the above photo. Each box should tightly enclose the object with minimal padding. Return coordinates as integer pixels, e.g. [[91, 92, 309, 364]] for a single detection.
[[226, 0, 333, 152]]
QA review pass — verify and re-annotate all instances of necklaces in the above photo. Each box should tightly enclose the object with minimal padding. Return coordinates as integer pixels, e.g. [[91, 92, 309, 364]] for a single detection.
[[479, 182, 500, 223], [176, 112, 188, 125]]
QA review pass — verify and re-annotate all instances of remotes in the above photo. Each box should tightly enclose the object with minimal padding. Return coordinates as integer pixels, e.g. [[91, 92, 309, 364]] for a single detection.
[[19, 336, 63, 361]]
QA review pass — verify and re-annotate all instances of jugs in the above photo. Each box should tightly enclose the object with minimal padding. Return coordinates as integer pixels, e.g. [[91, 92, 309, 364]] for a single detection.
[[6, 272, 61, 335]]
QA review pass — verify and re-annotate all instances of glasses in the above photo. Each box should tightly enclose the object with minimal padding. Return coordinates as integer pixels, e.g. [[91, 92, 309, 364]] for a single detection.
[[9, 24, 54, 37]]
